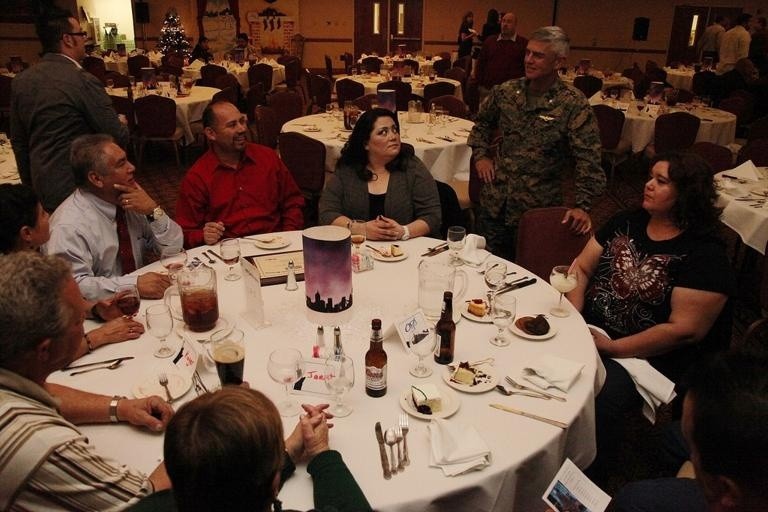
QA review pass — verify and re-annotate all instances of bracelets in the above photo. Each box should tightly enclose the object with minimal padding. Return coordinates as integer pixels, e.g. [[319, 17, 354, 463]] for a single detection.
[[281, 448, 297, 477], [109, 395, 123, 423]]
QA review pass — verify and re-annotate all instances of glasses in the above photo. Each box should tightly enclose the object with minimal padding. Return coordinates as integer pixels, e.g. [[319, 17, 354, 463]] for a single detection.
[[69, 31, 88, 39]]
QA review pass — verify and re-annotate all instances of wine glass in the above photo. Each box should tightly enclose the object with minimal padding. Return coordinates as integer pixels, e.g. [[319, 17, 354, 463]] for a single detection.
[[145, 303, 178, 359], [196, 56, 256, 72], [348, 220, 366, 252], [325, 99, 450, 135], [486, 295, 517, 349], [221, 238, 242, 281], [320, 355, 356, 418], [550, 266, 579, 316], [408, 320, 438, 377], [446, 227, 466, 267], [106, 75, 196, 98], [350, 53, 433, 87], [559, 62, 720, 118], [267, 348, 308, 416], [483, 261, 508, 300]]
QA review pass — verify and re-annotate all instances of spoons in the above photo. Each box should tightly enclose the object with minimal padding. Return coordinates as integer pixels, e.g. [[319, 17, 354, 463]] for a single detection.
[[202, 251, 215, 264], [496, 383, 550, 400], [385, 425, 397, 475], [69, 359, 124, 375]]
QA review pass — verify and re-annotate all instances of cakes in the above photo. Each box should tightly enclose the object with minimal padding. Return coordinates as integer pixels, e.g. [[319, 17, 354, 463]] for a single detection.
[[469, 299, 487, 316], [412, 383, 441, 414], [515, 315, 549, 336], [390, 244, 403, 257], [450, 363, 477, 387]]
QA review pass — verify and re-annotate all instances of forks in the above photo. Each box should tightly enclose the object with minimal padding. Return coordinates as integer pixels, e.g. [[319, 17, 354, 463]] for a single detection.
[[392, 425, 404, 473], [398, 411, 410, 466], [505, 375, 570, 403], [156, 372, 174, 403]]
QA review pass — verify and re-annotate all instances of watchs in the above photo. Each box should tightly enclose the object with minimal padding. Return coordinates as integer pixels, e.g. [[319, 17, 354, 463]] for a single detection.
[[402, 226, 410, 241]]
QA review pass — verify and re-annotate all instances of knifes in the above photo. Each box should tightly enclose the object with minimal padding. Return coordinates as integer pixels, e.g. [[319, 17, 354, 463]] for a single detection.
[[374, 421, 392, 480], [420, 242, 447, 256], [495, 278, 537, 295], [490, 402, 568, 430], [208, 249, 222, 260], [61, 356, 134, 370]]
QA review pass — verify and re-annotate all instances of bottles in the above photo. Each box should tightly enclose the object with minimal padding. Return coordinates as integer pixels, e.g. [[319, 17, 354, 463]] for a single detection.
[[432, 291, 455, 364], [285, 260, 299, 291], [364, 320, 388, 398], [315, 324, 329, 358], [329, 326, 346, 362]]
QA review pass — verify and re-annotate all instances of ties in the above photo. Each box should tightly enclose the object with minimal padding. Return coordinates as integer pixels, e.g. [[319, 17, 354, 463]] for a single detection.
[[115, 209, 137, 277]]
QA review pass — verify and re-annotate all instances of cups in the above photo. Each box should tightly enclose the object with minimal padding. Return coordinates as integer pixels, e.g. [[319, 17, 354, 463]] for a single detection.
[[114, 282, 141, 319], [210, 328, 246, 388], [160, 248, 188, 273]]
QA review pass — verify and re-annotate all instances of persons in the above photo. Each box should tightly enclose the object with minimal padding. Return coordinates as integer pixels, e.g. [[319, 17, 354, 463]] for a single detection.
[[10, 12, 128, 216], [695, 9, 768, 106], [466, 26, 606, 267], [174, 97, 304, 247], [456, 10, 530, 108], [1, 133, 182, 360], [1, 251, 172, 512], [223, 33, 257, 62], [189, 36, 214, 63], [124, 386, 373, 511], [564, 146, 735, 403], [317, 107, 440, 241]]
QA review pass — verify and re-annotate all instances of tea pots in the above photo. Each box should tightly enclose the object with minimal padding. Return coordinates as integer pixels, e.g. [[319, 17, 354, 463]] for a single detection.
[[418, 258, 468, 317], [161, 268, 219, 333]]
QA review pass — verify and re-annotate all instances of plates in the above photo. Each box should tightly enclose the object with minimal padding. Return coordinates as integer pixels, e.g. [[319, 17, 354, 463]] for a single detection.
[[400, 384, 461, 421], [130, 369, 193, 405], [175, 315, 235, 342], [442, 361, 501, 394], [370, 250, 407, 262], [509, 310, 559, 341], [460, 296, 493, 324], [254, 235, 290, 250]]
